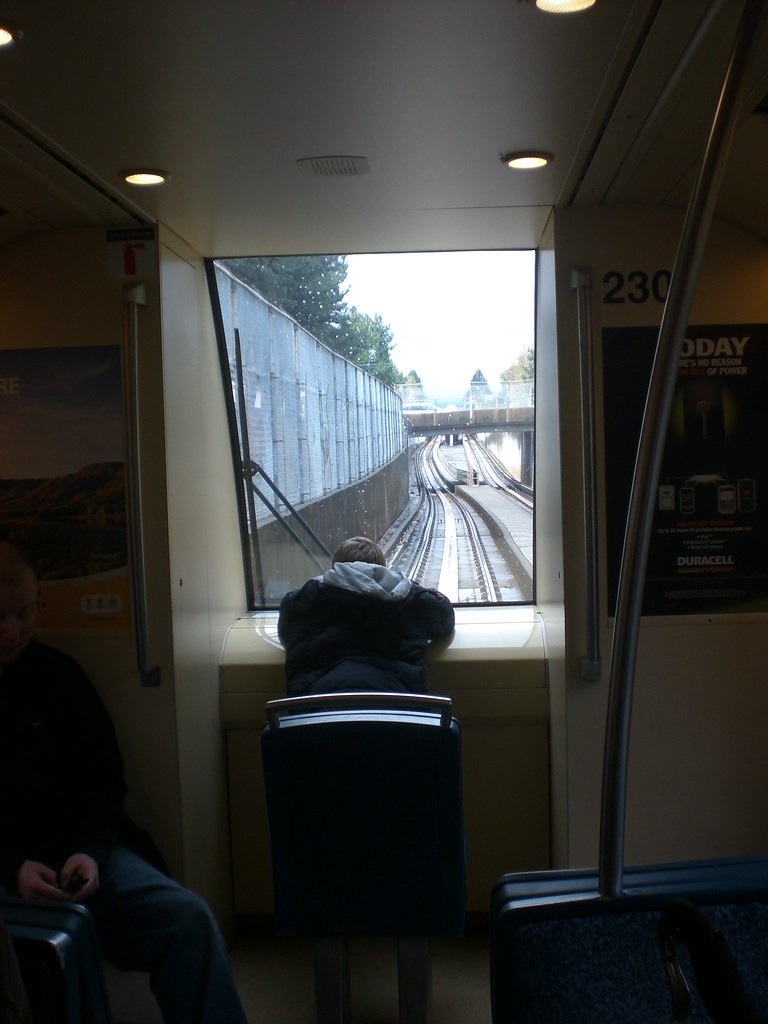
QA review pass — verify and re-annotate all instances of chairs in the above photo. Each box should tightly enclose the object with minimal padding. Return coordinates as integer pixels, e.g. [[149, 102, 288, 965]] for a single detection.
[[262, 690, 470, 1024]]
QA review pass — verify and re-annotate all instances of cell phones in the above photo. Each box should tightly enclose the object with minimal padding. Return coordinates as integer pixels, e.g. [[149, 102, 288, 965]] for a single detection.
[[55, 877, 92, 895]]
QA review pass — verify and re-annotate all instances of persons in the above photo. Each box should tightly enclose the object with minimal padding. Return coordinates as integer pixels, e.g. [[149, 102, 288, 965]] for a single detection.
[[277, 536, 456, 698], [1, 537, 250, 1024]]
